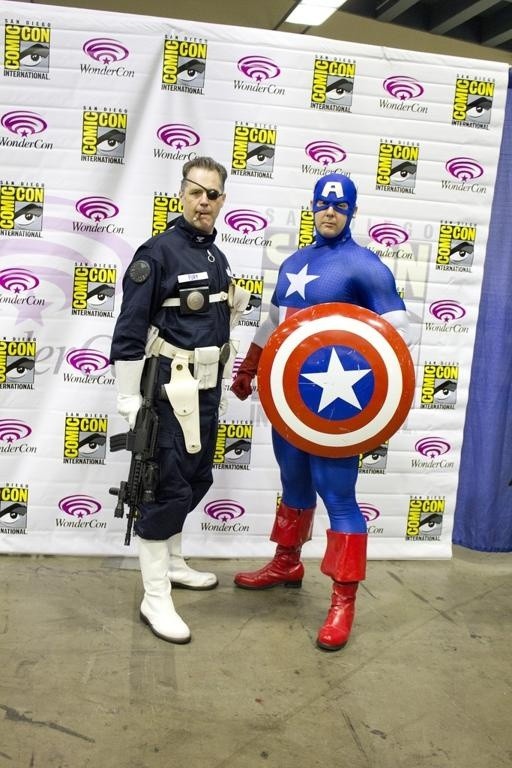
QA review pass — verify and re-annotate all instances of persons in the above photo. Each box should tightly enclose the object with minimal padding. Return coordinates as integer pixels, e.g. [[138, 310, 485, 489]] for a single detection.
[[227, 172, 415, 654], [107, 155, 243, 645]]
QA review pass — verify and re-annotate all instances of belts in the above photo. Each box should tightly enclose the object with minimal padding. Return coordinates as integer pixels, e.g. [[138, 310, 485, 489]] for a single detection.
[[151, 338, 229, 365]]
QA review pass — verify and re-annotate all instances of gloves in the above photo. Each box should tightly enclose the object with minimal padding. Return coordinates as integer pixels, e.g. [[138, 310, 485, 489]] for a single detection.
[[230, 342, 262, 400], [115, 357, 145, 431]]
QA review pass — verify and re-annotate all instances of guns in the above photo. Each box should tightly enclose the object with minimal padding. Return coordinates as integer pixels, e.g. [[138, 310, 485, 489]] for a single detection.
[[110, 357, 159, 545]]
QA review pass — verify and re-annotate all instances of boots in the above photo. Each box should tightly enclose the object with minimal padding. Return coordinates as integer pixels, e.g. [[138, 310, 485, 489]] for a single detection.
[[166, 532, 218, 590], [316, 529, 367, 649], [135, 536, 191, 643], [234, 502, 316, 590]]
